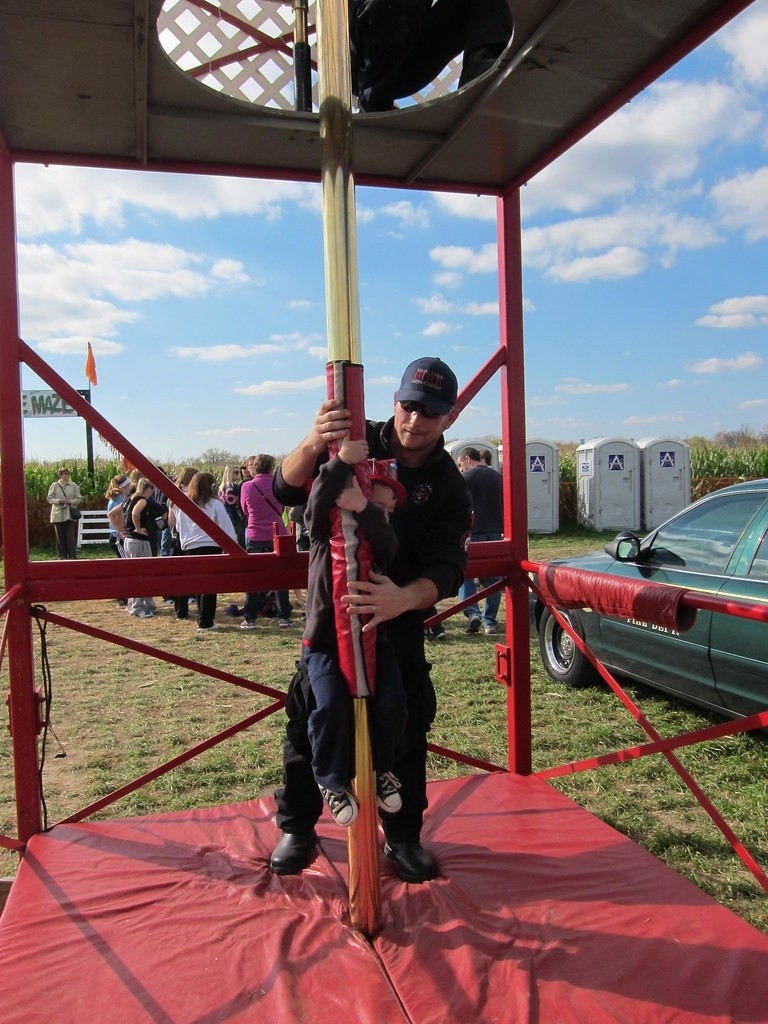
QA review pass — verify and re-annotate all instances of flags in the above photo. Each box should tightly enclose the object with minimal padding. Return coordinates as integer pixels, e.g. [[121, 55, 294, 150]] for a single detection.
[[85, 342, 98, 386]]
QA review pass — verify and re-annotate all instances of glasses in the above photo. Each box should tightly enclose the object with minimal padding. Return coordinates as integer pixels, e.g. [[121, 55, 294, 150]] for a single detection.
[[233, 471, 238, 474], [400, 399, 441, 419], [63, 472, 70, 475]]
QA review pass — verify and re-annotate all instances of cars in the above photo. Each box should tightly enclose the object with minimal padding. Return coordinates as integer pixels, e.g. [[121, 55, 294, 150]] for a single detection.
[[529, 478, 768, 723]]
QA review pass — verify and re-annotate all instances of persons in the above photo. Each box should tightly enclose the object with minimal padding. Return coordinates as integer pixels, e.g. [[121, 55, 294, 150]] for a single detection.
[[47, 467, 82, 559], [104, 475, 130, 604], [167, 467, 200, 619], [126, 469, 142, 497], [474, 449, 505, 593], [240, 459, 250, 480], [107, 478, 157, 618], [288, 505, 310, 551], [424, 605, 446, 643], [218, 464, 247, 550], [457, 447, 505, 633], [304, 430, 407, 827], [168, 472, 238, 632], [154, 466, 176, 557], [236, 456, 247, 527], [271, 355, 475, 883], [240, 454, 294, 628]]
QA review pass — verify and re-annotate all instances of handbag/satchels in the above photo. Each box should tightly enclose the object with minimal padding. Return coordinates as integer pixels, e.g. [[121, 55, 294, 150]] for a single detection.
[[70, 507, 83, 520]]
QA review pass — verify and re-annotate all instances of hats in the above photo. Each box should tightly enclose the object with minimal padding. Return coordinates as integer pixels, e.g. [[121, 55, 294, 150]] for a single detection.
[[366, 457, 405, 508], [393, 353, 457, 414]]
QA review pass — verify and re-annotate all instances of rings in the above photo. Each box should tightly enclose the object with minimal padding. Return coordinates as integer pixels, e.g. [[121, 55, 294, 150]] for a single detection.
[[372, 605, 376, 614]]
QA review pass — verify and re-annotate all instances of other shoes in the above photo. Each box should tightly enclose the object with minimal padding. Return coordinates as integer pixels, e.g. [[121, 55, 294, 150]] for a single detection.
[[485, 624, 500, 634], [136, 609, 153, 617], [240, 619, 256, 630], [466, 614, 481, 633], [198, 623, 217, 632], [278, 618, 292, 626], [433, 628, 446, 640]]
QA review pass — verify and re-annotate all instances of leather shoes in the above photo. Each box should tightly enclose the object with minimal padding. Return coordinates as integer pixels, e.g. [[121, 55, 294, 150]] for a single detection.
[[269, 828, 316, 876], [384, 838, 437, 883]]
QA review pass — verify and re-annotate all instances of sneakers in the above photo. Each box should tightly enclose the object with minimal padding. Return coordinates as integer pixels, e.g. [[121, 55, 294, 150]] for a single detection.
[[372, 768, 404, 813], [317, 781, 359, 829]]
